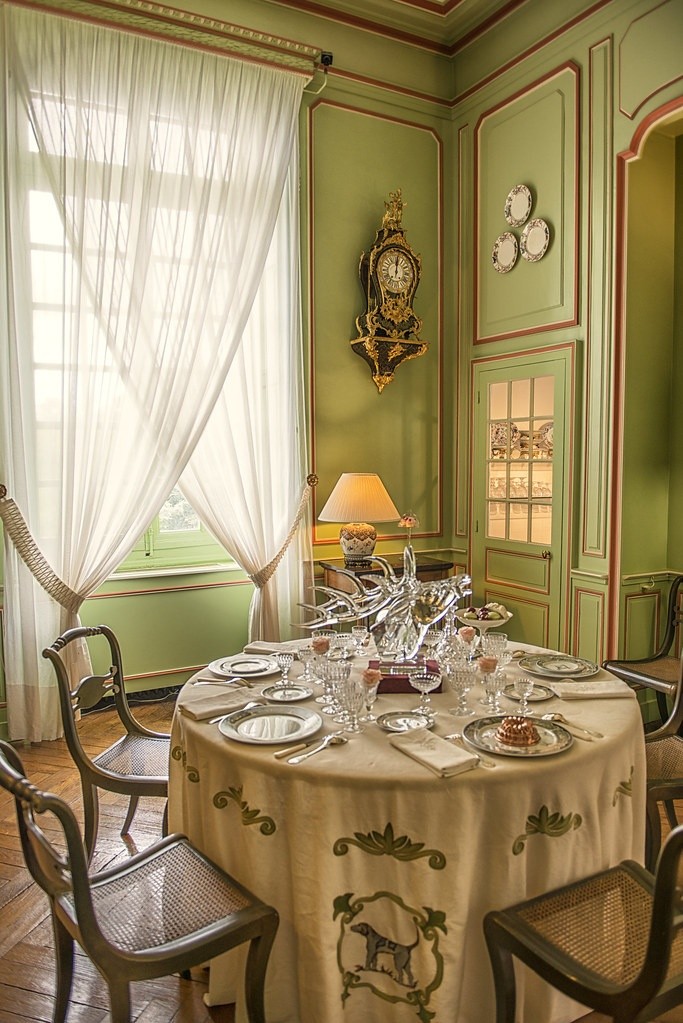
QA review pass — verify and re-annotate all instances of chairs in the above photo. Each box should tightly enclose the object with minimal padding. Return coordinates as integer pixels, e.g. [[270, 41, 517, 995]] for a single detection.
[[645, 638, 683, 874], [482, 822, 683, 1023], [0, 740, 281, 1023], [602, 574, 683, 726], [42, 625, 172, 870]]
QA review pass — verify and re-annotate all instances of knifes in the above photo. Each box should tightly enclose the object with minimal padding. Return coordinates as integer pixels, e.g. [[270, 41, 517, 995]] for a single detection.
[[274, 731, 343, 757]]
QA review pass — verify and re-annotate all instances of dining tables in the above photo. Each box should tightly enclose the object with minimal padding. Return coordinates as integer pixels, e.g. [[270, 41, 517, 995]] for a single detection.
[[168, 628, 648, 1023]]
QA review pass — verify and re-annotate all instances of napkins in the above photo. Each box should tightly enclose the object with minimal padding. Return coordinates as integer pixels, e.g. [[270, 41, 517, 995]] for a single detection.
[[243, 641, 303, 660], [387, 724, 481, 780], [178, 686, 267, 722], [549, 679, 636, 700]]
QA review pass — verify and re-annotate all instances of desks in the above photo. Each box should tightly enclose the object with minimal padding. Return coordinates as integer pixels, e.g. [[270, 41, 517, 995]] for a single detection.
[[319, 554, 455, 631]]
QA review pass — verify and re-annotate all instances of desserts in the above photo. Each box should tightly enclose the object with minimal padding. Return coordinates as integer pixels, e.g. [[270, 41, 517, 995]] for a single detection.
[[495, 715, 539, 746]]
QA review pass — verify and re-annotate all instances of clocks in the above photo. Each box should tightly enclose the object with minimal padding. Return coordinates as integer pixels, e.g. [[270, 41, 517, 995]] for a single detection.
[[350, 186, 431, 394]]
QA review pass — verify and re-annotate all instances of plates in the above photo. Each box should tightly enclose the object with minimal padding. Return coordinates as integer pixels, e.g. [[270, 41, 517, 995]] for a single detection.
[[330, 647, 353, 659], [261, 684, 313, 701], [218, 705, 322, 744], [463, 714, 573, 757], [456, 607, 513, 627], [208, 654, 281, 677], [472, 653, 481, 658], [502, 683, 554, 701], [517, 653, 600, 678], [377, 711, 435, 732]]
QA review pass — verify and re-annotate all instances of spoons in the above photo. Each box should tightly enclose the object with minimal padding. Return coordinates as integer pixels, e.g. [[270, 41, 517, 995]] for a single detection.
[[542, 713, 604, 739], [288, 734, 348, 764]]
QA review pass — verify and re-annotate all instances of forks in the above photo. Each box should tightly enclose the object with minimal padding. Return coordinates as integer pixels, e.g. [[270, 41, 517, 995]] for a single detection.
[[193, 678, 254, 688], [208, 701, 267, 724], [445, 734, 495, 767]]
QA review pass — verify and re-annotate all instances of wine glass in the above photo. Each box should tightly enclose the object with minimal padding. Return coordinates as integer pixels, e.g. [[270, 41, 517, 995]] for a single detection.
[[424, 629, 443, 660], [296, 626, 379, 733], [407, 671, 441, 717], [512, 679, 533, 716], [275, 652, 295, 686], [445, 631, 513, 716]]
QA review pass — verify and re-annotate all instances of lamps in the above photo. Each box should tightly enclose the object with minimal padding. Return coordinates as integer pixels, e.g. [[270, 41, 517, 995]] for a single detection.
[[317, 472, 401, 565]]
